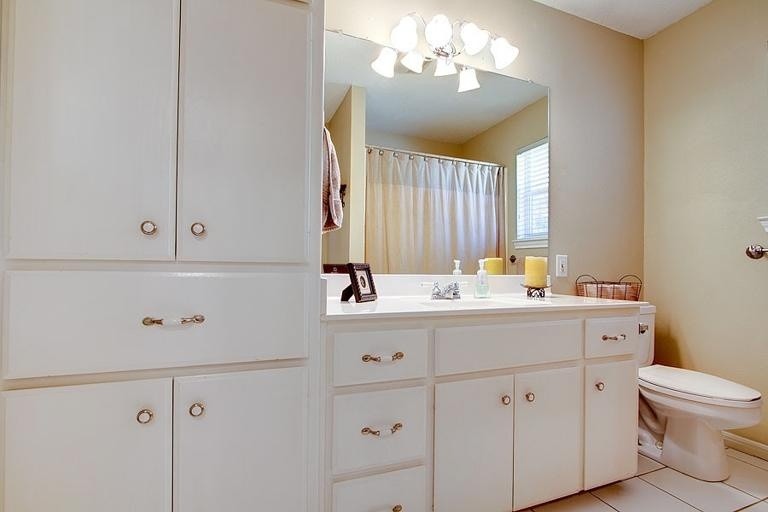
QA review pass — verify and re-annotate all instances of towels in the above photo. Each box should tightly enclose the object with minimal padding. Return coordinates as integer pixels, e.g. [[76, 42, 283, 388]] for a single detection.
[[322, 128, 344, 236]]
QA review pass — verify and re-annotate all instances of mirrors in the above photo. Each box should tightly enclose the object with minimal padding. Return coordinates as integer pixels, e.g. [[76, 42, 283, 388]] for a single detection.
[[325, 26, 551, 275]]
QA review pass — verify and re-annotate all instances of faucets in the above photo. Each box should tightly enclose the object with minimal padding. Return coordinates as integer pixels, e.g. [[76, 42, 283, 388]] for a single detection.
[[442, 281, 459, 300]]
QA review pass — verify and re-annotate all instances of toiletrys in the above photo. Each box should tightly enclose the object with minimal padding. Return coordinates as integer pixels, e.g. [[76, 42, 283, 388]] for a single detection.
[[472, 259, 490, 298], [453, 259, 461, 274]]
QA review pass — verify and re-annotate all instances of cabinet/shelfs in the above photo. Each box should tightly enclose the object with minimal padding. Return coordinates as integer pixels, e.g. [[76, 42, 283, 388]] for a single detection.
[[1, 362, 318, 510], [2, 264, 319, 387], [586, 308, 640, 490], [327, 322, 434, 512], [437, 320, 585, 510], [0, 1, 321, 266]]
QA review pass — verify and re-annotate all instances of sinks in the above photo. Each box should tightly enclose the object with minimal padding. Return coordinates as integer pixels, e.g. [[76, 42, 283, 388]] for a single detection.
[[417, 298, 517, 309]]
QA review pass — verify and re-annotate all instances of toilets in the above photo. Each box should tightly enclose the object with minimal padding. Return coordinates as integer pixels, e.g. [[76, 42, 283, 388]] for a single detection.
[[637, 301, 764, 483]]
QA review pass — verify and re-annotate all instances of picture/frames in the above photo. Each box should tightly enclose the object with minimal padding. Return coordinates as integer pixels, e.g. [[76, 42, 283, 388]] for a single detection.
[[346, 262, 378, 303]]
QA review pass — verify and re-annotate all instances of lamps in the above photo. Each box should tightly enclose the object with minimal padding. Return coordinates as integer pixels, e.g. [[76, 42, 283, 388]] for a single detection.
[[387, 12, 519, 70], [367, 46, 481, 93]]
